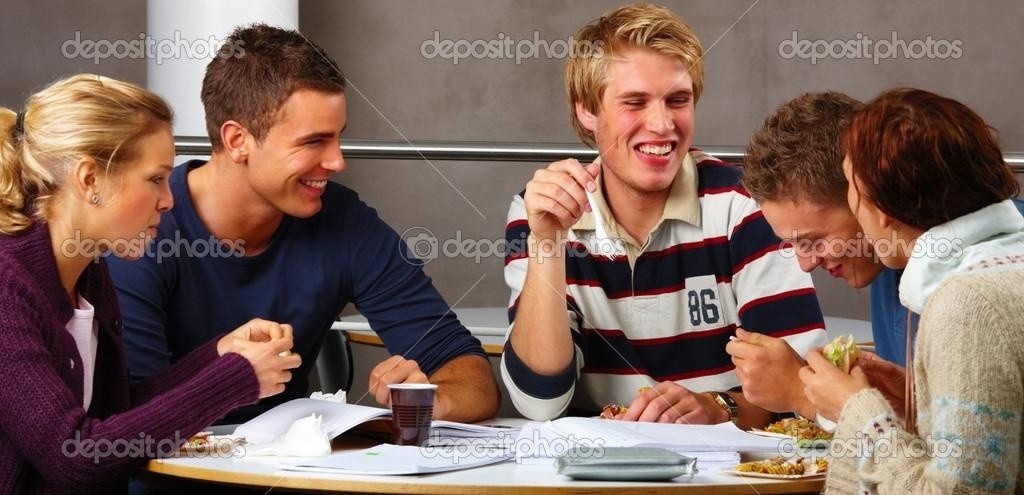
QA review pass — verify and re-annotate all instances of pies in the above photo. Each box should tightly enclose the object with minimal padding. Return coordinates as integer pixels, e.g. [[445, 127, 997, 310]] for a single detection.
[[823, 335, 856, 375]]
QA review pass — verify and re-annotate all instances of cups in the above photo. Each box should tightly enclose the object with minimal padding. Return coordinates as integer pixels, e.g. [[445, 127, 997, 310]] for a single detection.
[[388, 384, 437, 448]]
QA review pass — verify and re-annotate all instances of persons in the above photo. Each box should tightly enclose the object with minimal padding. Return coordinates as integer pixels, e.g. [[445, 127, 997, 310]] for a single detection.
[[100, 23, 503, 425], [0, 71, 305, 495], [820, 77, 1024, 495], [723, 88, 924, 434], [496, 0, 832, 424]]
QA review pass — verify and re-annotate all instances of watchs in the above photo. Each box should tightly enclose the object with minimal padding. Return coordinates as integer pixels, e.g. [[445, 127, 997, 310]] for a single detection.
[[705, 388, 738, 424]]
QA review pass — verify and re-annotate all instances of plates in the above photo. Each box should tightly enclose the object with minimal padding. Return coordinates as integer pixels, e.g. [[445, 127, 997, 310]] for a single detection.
[[729, 463, 837, 482], [750, 429, 842, 447], [552, 445, 700, 481]]
